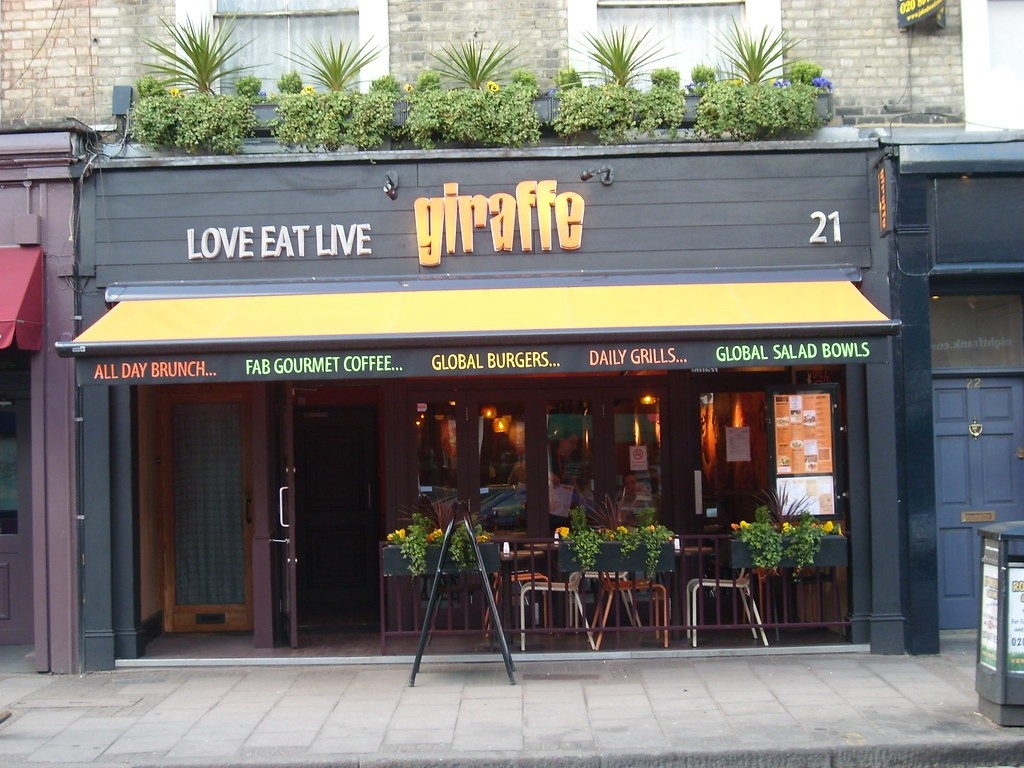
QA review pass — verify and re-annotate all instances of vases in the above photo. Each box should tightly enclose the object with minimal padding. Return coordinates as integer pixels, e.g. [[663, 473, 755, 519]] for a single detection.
[[731, 533, 848, 569], [559, 540, 675, 573], [128, 93, 833, 135], [382, 542, 500, 576]]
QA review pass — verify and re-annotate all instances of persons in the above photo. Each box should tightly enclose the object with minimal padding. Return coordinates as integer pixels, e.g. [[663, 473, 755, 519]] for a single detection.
[[507, 460, 527, 530], [617, 470, 661, 527], [550, 427, 587, 534]]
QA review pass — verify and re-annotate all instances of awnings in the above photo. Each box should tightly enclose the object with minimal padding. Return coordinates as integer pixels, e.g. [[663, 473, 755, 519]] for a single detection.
[[53, 281, 902, 388], [0, 247, 44, 350]]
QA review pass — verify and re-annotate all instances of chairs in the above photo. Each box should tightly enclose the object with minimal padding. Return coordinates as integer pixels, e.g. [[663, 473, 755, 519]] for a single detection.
[[481, 530, 843, 652]]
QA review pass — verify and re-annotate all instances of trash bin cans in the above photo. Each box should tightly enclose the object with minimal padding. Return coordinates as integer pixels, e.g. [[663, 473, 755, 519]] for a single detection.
[[975, 520, 1024, 725]]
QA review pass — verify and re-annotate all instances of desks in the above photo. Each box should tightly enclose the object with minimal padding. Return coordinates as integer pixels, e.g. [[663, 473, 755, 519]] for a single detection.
[[483, 543, 712, 650]]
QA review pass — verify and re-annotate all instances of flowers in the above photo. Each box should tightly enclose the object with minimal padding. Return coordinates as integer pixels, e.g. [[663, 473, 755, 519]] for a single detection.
[[125, 8, 835, 155], [385, 492, 494, 576], [730, 481, 844, 582], [558, 487, 675, 581]]
